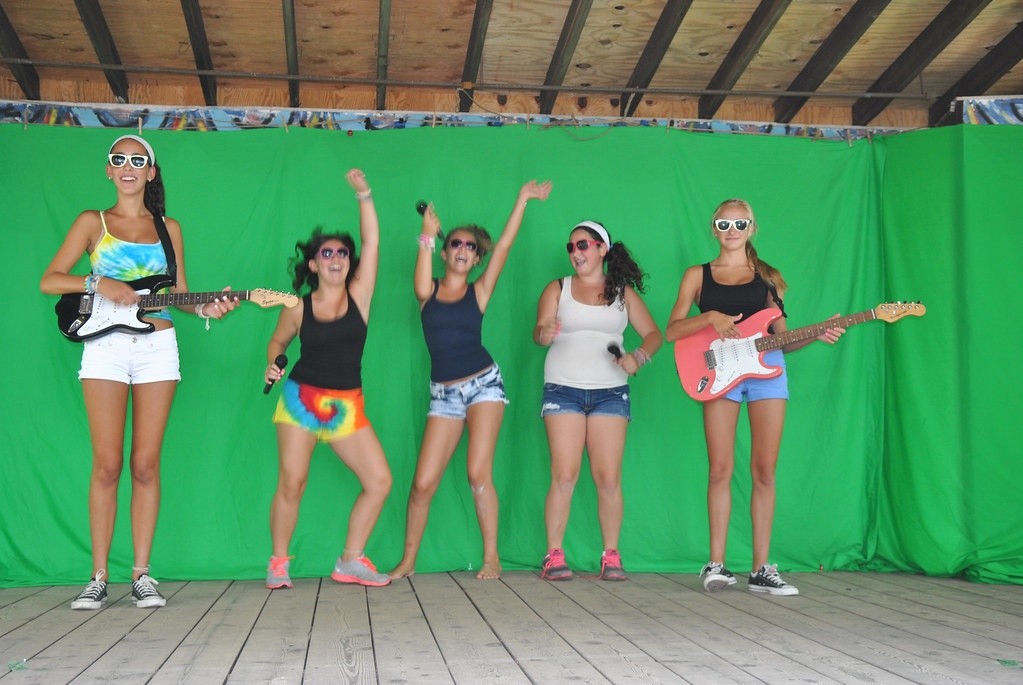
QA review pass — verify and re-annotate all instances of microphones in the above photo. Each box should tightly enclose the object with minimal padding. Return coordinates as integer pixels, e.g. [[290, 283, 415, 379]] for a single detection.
[[608, 344, 637, 377], [416, 202, 444, 241], [263, 355, 288, 394]]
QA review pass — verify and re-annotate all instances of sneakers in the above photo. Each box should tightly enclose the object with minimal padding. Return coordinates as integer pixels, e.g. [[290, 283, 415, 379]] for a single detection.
[[331, 554, 392, 587], [131, 573, 166, 608], [597, 549, 627, 581], [266, 557, 293, 588], [699, 562, 737, 593], [748, 564, 800, 595], [71, 569, 107, 609], [540, 548, 573, 580]]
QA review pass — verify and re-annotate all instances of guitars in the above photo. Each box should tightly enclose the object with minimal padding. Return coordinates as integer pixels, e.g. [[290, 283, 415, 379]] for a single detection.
[[673, 299, 930, 402], [55, 273, 301, 345]]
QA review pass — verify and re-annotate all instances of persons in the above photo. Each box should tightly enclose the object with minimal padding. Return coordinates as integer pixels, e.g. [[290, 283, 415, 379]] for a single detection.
[[666, 198, 846, 597], [265, 168, 392, 590], [389, 179, 551, 581], [533, 221, 662, 582], [40, 136, 241, 609]]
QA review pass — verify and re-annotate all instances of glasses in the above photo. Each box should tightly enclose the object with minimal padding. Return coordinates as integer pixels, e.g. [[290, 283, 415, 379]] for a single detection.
[[566, 240, 602, 253], [108, 154, 149, 169], [315, 248, 349, 259], [715, 219, 752, 232], [446, 239, 476, 250]]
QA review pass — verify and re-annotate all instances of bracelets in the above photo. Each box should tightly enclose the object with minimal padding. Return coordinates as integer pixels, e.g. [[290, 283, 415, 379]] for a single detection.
[[633, 348, 649, 367], [195, 303, 210, 319], [84, 274, 103, 295], [518, 199, 527, 207], [419, 234, 435, 248], [357, 190, 371, 201]]
